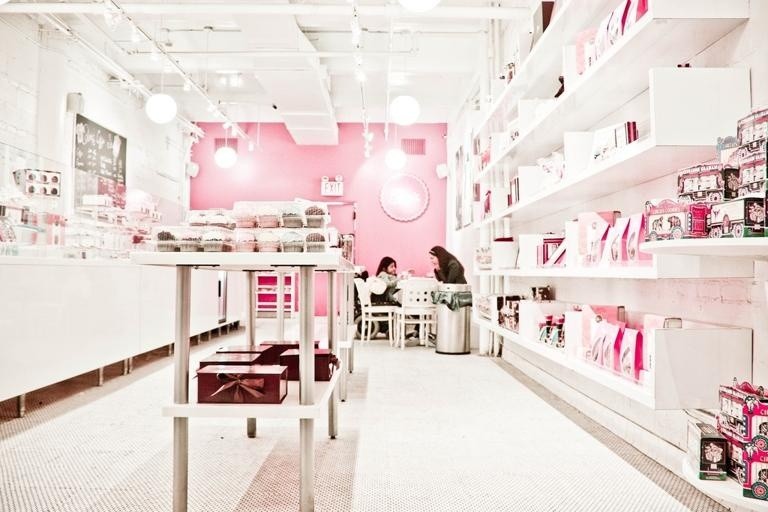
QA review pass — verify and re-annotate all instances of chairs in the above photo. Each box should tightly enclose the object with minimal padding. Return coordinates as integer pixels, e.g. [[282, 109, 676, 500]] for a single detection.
[[353, 276, 401, 345], [396, 277, 444, 352]]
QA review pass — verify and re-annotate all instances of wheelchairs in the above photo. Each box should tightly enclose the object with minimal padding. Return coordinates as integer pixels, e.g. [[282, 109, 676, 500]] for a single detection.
[[338, 266, 401, 345]]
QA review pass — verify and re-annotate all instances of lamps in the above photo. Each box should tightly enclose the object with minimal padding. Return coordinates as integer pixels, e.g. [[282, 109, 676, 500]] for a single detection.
[[321, 175, 346, 198], [143, 52, 179, 127], [213, 118, 241, 175]]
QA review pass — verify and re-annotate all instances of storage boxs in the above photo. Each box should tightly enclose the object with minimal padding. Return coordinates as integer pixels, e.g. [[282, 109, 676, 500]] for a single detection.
[[196, 341, 339, 404]]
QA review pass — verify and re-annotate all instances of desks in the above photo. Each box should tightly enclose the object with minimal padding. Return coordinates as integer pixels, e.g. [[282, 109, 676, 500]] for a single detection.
[[275, 262, 365, 403], [395, 280, 405, 342], [134, 249, 356, 512]]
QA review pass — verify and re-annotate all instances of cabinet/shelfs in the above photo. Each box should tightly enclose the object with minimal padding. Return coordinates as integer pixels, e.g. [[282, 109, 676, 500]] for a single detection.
[[450, 2, 768, 512], [254, 270, 296, 323], [1, 265, 181, 402], [189, 264, 245, 339]]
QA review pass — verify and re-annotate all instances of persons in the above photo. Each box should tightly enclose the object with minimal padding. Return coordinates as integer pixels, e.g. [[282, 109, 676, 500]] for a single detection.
[[372, 256, 401, 339], [406, 246, 467, 340]]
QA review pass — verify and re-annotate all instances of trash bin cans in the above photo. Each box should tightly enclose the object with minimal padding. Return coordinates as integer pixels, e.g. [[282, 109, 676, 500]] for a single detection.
[[431, 283, 474, 354]]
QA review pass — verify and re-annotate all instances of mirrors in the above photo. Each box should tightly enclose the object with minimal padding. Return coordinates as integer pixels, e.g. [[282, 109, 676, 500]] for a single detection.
[[378, 172, 431, 224]]
